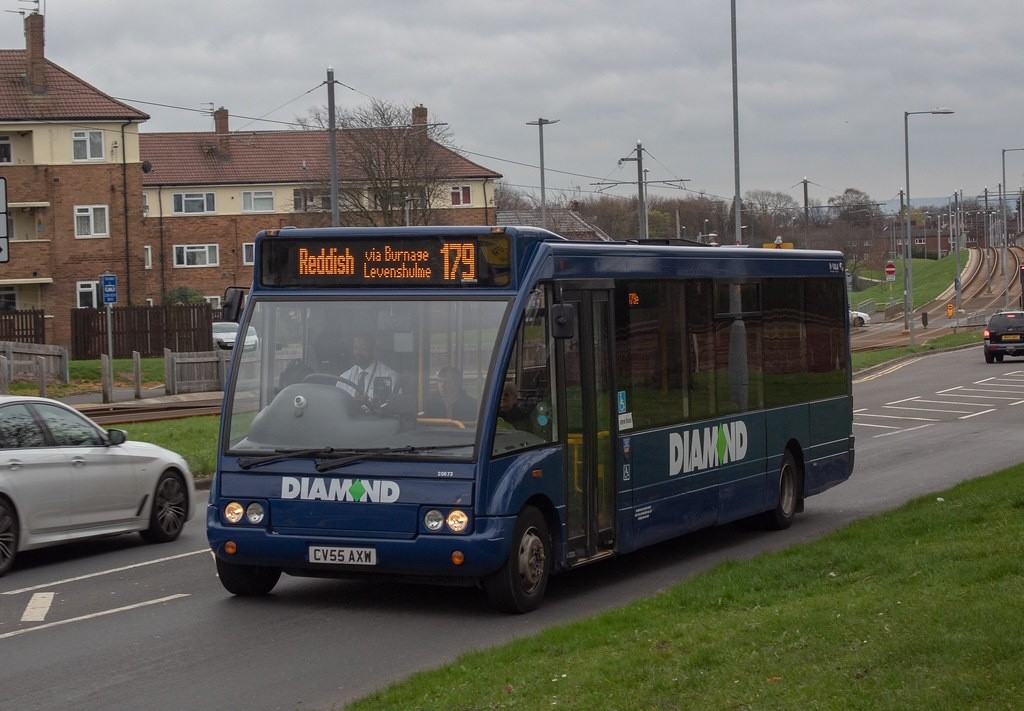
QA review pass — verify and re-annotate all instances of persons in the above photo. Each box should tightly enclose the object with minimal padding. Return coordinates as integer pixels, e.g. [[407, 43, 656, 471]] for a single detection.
[[335, 333, 403, 413], [495, 382, 533, 434], [425, 366, 478, 428]]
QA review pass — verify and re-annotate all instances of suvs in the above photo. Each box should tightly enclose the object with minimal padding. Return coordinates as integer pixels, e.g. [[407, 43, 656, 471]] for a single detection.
[[983, 309, 1024, 363]]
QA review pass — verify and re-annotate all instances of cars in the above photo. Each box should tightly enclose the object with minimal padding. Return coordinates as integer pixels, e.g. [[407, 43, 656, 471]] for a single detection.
[[849, 310, 870, 327], [211, 321, 259, 352], [0, 394, 197, 574]]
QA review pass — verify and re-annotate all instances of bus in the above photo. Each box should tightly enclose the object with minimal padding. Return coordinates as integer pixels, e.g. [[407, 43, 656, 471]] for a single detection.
[[205, 225, 856, 616]]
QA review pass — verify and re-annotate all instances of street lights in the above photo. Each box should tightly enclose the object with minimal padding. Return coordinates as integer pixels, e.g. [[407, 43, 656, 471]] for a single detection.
[[904, 108, 955, 314], [642, 168, 650, 239], [526, 117, 560, 229]]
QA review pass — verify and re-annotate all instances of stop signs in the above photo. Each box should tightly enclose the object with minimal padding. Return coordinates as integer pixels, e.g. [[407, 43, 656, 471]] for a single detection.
[[885, 263, 896, 275]]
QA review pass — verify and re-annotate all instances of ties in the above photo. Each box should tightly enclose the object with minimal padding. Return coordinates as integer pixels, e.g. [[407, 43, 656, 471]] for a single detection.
[[357, 372, 368, 394]]
